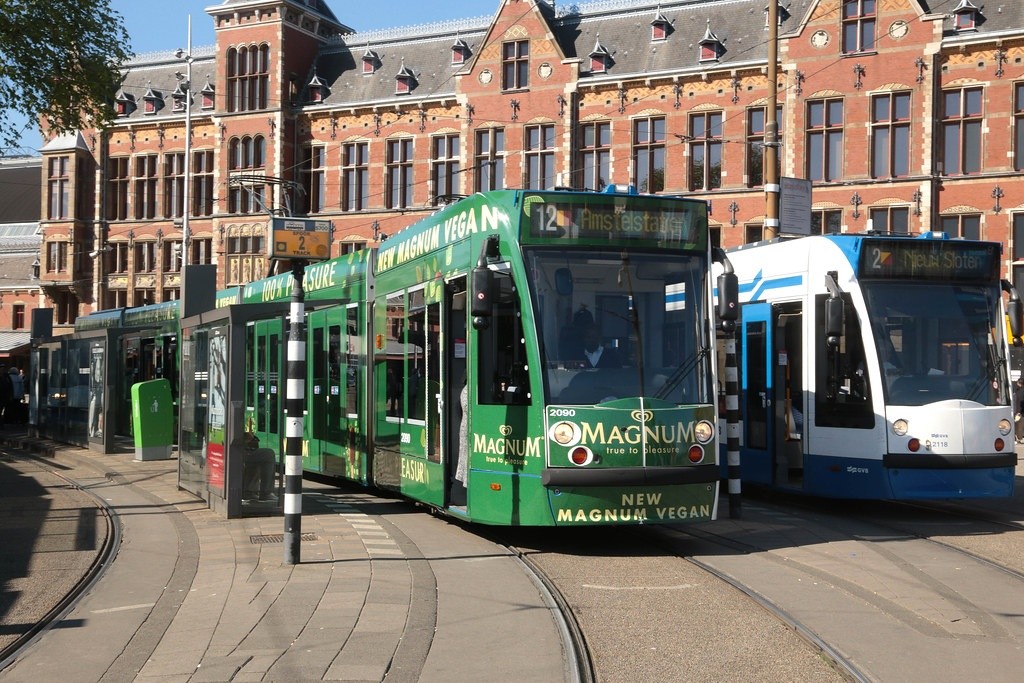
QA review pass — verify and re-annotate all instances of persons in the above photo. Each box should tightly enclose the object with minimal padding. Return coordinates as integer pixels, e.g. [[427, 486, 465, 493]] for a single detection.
[[244, 448, 279, 499], [572, 323, 623, 368], [454, 370, 469, 488], [0, 364, 25, 429], [857, 339, 896, 376], [1014, 378, 1024, 444]]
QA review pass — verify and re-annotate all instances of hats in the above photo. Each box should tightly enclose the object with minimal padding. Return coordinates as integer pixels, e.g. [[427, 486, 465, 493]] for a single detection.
[[8, 367, 18, 374]]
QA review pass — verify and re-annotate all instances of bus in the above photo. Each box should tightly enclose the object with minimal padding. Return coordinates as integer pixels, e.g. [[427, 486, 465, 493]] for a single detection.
[[628, 232, 1019, 503], [72, 186, 723, 527]]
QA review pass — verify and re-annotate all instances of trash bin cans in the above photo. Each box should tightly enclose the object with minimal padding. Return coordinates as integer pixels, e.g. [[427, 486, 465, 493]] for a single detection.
[[131, 378, 175, 461]]
